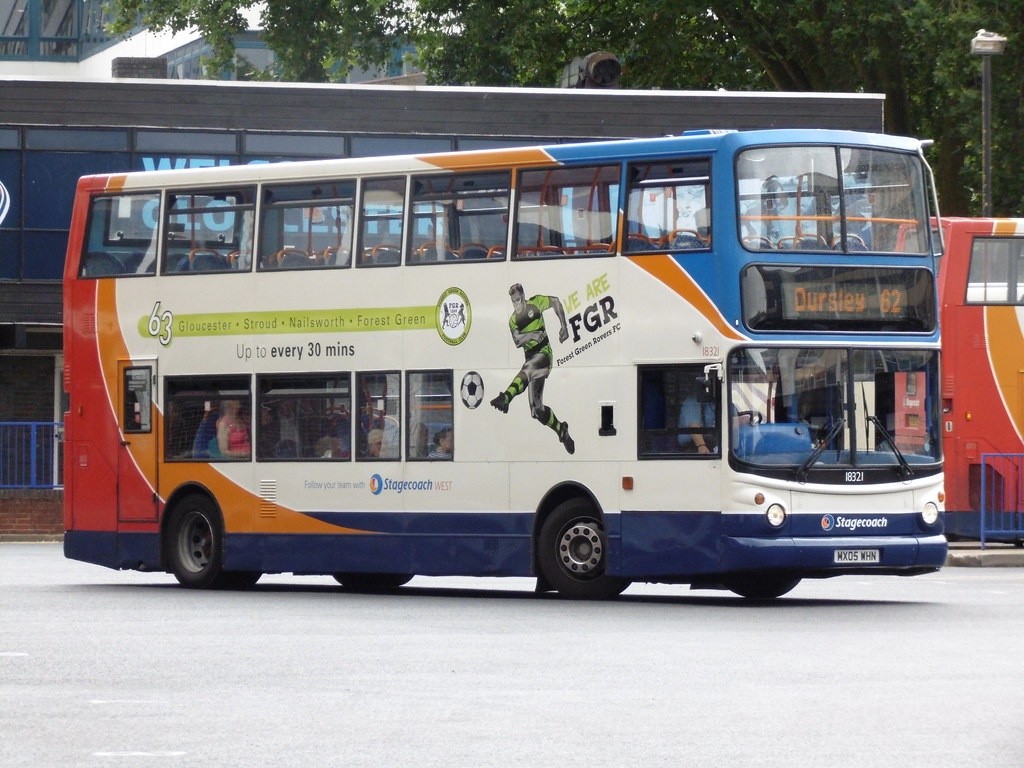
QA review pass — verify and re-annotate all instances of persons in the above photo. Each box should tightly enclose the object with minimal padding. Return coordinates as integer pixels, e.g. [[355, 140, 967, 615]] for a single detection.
[[678, 380, 740, 453], [216, 395, 453, 459]]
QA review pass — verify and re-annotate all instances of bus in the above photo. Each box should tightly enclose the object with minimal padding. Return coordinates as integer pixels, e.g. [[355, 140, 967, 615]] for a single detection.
[[59, 129, 951, 603], [889, 217, 1024, 546]]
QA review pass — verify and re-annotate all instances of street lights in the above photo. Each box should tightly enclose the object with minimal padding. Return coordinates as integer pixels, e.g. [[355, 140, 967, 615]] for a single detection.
[[973, 29, 1009, 212]]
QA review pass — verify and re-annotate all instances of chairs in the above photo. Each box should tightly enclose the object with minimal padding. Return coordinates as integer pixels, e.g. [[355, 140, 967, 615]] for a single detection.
[[87, 230, 873, 275]]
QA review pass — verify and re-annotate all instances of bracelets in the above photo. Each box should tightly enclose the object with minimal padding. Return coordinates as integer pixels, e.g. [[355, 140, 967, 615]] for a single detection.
[[697, 443, 705, 448]]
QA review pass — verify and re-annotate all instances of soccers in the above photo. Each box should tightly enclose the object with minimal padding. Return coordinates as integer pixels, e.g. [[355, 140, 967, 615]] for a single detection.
[[460, 371, 485, 410]]
